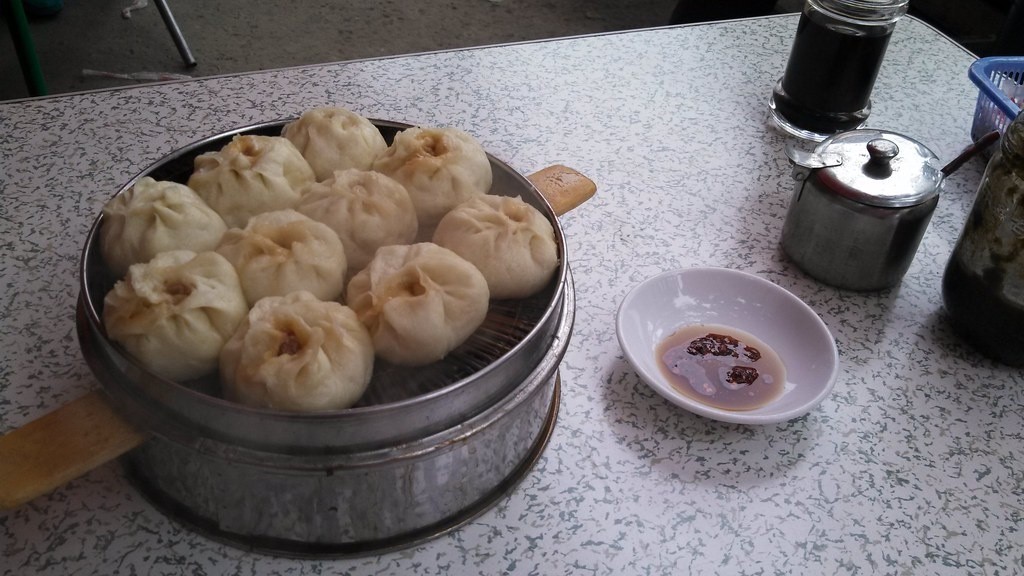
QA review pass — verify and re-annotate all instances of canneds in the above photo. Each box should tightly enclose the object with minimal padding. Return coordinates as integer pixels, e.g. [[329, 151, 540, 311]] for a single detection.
[[941, 110, 1024, 372]]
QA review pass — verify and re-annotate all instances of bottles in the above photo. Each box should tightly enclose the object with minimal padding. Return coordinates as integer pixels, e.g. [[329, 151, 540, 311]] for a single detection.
[[770, 0, 909, 142]]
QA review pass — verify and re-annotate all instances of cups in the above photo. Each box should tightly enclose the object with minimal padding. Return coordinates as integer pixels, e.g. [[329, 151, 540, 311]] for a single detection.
[[783, 128, 944, 293], [943, 111, 1024, 361]]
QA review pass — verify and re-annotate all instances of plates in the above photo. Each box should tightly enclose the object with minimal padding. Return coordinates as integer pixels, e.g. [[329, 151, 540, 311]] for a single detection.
[[616, 268, 839, 424]]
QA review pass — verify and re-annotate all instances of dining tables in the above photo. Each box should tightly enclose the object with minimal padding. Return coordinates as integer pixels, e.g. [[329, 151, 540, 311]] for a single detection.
[[0, 12, 1024, 576]]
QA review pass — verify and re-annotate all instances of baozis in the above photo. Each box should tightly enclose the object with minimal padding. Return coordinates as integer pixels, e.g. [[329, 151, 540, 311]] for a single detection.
[[95, 107, 558, 409]]
